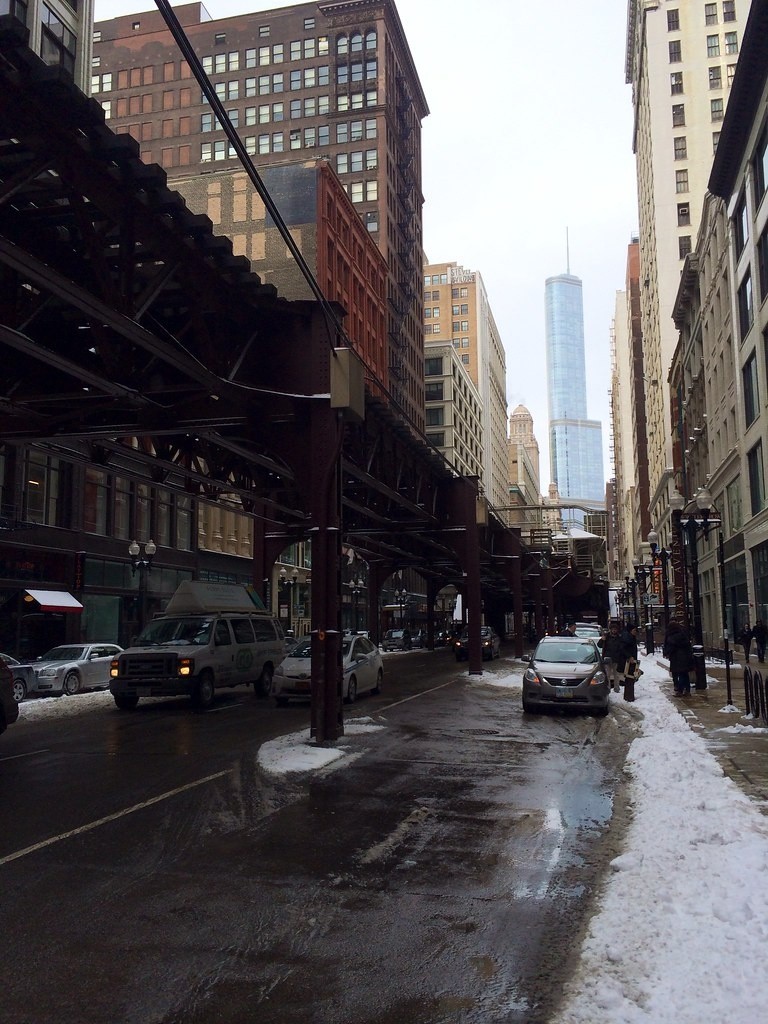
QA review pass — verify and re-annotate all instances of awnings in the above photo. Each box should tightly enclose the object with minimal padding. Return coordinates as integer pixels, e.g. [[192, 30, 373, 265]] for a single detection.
[[25, 589, 84, 613]]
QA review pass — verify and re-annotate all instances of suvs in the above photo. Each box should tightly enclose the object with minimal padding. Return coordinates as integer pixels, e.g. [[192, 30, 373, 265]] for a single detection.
[[434, 630, 453, 647], [455, 625, 501, 661], [382, 629, 413, 652], [410, 630, 426, 649]]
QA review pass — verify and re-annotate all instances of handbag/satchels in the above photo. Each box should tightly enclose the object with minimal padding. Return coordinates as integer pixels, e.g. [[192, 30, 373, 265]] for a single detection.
[[688, 657, 696, 671]]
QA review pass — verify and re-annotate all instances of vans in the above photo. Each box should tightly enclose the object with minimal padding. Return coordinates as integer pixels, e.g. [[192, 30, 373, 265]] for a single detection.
[[109, 578, 284, 709]]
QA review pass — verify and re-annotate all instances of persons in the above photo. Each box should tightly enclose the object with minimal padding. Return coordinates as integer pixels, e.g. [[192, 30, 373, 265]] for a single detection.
[[752, 619, 768, 663], [739, 623, 753, 663], [663, 621, 695, 697], [616, 625, 638, 702], [597, 623, 622, 693], [559, 622, 578, 637], [401, 626, 427, 651]]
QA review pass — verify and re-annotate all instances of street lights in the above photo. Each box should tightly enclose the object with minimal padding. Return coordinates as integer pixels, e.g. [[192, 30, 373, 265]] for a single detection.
[[670, 487, 712, 691], [647, 529, 671, 659], [632, 558, 649, 644], [281, 566, 299, 637], [129, 538, 156, 633], [348, 580, 364, 630], [614, 569, 639, 635], [395, 589, 407, 629]]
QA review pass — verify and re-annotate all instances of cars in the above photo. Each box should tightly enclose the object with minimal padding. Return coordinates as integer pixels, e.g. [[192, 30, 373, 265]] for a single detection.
[[0, 654, 38, 703], [574, 623, 614, 681], [30, 643, 124, 696], [271, 634, 384, 707], [284, 637, 298, 655], [523, 623, 535, 634], [521, 635, 611, 717]]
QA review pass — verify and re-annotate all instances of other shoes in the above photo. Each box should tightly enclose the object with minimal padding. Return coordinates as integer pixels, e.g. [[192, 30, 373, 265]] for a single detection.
[[674, 693, 683, 697], [684, 692, 691, 696], [746, 660, 749, 663]]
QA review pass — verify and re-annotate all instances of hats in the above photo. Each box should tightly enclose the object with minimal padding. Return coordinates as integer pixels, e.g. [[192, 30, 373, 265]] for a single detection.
[[626, 622, 636, 632], [568, 622, 576, 628]]
